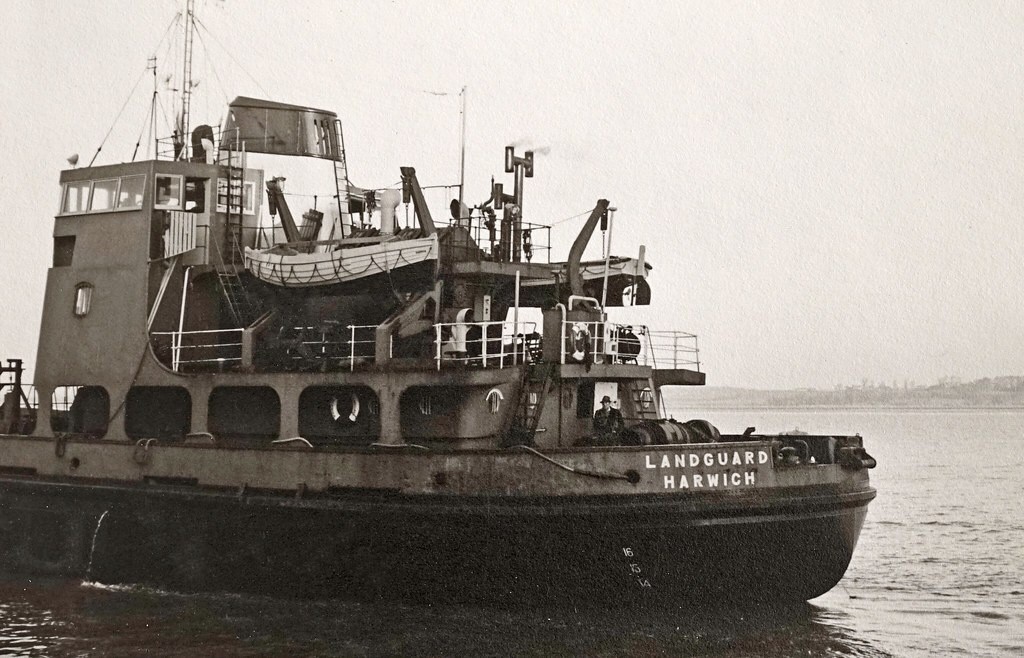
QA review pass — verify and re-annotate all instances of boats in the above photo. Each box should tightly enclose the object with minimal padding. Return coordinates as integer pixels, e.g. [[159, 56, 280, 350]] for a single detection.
[[0, 0, 878, 614]]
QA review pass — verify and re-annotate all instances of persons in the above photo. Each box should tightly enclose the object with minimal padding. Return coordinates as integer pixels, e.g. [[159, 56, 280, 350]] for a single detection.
[[595, 396, 624, 446], [122, 185, 139, 207]]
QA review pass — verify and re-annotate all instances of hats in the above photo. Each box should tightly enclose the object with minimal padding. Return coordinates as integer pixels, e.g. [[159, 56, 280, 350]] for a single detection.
[[600, 396, 612, 403]]
